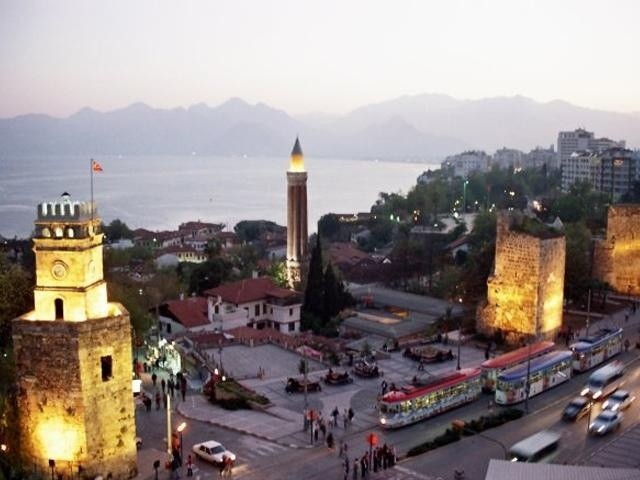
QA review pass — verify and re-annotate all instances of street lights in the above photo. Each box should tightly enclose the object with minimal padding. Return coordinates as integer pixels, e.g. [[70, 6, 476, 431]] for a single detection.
[[452, 419, 508, 460]]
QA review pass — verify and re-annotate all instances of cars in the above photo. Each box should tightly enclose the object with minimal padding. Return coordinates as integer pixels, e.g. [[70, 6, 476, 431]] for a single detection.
[[562, 397, 590, 421], [193, 440, 236, 466], [588, 390, 634, 436]]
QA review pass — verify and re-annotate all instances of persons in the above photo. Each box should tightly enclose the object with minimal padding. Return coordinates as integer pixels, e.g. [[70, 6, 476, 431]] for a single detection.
[[383, 344, 387, 352], [624, 338, 630, 352], [308, 407, 396, 480], [184, 454, 193, 477], [557, 326, 580, 347], [218, 454, 233, 479], [381, 380, 387, 395], [417, 356, 425, 371], [136, 353, 186, 410]]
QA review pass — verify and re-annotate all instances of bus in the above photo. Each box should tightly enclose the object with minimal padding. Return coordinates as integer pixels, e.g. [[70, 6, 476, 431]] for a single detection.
[[568, 327, 623, 372], [507, 430, 561, 463], [377, 368, 483, 430], [580, 361, 625, 401], [483, 341, 573, 406]]
